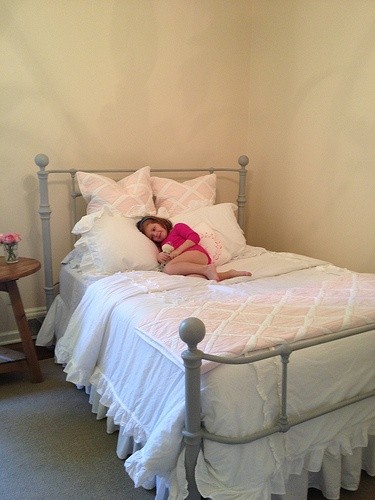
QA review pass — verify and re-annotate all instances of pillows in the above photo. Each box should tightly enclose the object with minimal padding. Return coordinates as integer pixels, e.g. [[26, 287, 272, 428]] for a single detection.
[[73, 163, 159, 223], [71, 211, 168, 278], [171, 200, 245, 257], [150, 173, 218, 220]]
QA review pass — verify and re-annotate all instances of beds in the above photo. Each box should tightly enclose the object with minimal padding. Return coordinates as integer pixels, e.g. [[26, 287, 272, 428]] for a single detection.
[[33, 154, 374, 500]]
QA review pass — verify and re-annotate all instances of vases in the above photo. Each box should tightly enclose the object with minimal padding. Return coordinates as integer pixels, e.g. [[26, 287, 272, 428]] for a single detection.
[[3, 232, 26, 262]]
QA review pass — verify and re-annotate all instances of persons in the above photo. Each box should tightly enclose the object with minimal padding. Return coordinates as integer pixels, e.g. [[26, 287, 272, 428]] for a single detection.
[[136, 215, 252, 281]]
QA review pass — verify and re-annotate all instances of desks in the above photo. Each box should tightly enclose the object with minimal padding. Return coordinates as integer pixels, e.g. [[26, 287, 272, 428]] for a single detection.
[[1, 253, 45, 382]]
